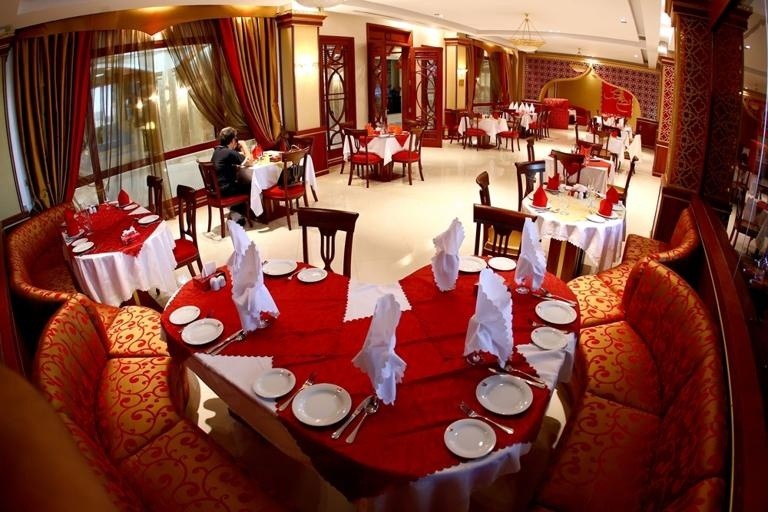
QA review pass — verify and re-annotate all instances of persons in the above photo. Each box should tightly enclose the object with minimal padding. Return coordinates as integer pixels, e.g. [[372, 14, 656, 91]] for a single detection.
[[209, 127, 254, 227]]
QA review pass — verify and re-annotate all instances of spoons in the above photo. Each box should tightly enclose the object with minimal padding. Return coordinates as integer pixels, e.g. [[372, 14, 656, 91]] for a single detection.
[[210, 332, 246, 356]]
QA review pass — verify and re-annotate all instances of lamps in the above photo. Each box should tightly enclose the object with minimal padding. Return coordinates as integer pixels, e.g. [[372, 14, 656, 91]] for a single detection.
[[296, 0, 347, 11], [509, 13, 547, 53], [569, 48, 588, 73]]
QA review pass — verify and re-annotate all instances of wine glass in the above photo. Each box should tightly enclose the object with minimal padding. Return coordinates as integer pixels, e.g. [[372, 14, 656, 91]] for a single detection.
[[560, 198, 569, 216], [104, 194, 111, 210], [516, 267, 530, 294]]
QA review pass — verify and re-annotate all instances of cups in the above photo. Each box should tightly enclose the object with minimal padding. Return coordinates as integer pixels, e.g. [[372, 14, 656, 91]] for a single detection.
[[553, 195, 560, 212]]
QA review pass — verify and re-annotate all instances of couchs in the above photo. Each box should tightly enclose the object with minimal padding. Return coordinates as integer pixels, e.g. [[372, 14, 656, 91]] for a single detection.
[[34, 298, 178, 464], [574, 105, 587, 126], [538, 357, 733, 512], [663, 478, 724, 511], [577, 255, 733, 416], [106, 305, 171, 357], [564, 274, 627, 328], [59, 411, 281, 512], [7, 202, 84, 356], [621, 209, 696, 260], [600, 230, 698, 301]]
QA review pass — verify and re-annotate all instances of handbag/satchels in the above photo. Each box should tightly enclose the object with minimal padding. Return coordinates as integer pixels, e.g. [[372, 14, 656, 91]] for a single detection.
[[277, 165, 305, 189]]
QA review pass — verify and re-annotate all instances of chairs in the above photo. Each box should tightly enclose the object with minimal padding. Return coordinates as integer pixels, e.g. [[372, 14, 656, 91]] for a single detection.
[[472, 203, 535, 265], [577, 142, 603, 157], [542, 110, 552, 138], [402, 119, 424, 168], [344, 127, 384, 188], [574, 122, 585, 153], [592, 129, 609, 160], [607, 156, 639, 208], [338, 121, 374, 173], [290, 144, 319, 210], [450, 110, 470, 146], [729, 188, 760, 255], [551, 150, 586, 185], [504, 107, 520, 131], [477, 171, 523, 260], [144, 175, 164, 219], [618, 133, 641, 175], [389, 124, 426, 186], [195, 159, 254, 239], [495, 117, 521, 152], [261, 145, 311, 230], [155, 184, 204, 295], [294, 206, 361, 277], [515, 160, 546, 212], [214, 144, 245, 156], [730, 164, 751, 207], [524, 111, 541, 142], [463, 113, 486, 151]]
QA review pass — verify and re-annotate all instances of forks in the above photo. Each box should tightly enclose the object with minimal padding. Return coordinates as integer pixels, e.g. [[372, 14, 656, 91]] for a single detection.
[[460, 403, 515, 434], [527, 317, 569, 334], [497, 359, 545, 384], [539, 288, 577, 304], [278, 378, 314, 411]]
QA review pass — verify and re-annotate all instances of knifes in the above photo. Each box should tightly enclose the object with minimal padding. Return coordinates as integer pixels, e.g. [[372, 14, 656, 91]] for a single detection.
[[345, 397, 380, 444], [331, 394, 374, 439], [532, 293, 576, 307], [205, 328, 243, 354], [488, 368, 545, 389], [287, 266, 307, 280]]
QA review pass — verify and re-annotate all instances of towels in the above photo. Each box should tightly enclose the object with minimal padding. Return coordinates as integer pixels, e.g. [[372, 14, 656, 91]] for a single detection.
[[352, 295, 405, 405], [517, 332, 575, 396], [618, 118, 625, 129], [226, 221, 255, 274], [118, 189, 128, 207], [519, 103, 525, 112], [546, 173, 560, 190], [533, 185, 547, 207], [493, 112, 498, 120], [514, 102, 519, 111], [530, 103, 534, 113], [432, 217, 464, 293], [525, 103, 529, 112], [599, 199, 612, 216], [232, 243, 277, 333], [612, 131, 618, 138], [514, 217, 546, 290], [463, 268, 513, 366], [67, 220, 78, 236], [509, 102, 514, 111], [606, 186, 617, 204], [64, 209, 75, 224], [580, 146, 591, 157], [610, 117, 615, 124]]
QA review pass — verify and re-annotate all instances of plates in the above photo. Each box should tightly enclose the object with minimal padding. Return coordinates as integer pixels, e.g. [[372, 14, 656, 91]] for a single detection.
[[488, 257, 516, 271], [529, 201, 552, 209], [262, 259, 297, 275], [138, 215, 160, 224], [444, 418, 497, 458], [612, 204, 623, 211], [168, 305, 200, 325], [292, 383, 352, 427], [181, 318, 224, 345], [475, 375, 534, 416], [72, 241, 94, 253], [71, 237, 88, 246], [585, 214, 605, 223], [531, 327, 568, 350], [253, 367, 296, 399], [595, 210, 618, 219], [123, 204, 139, 211], [535, 300, 578, 325], [458, 256, 487, 273], [297, 267, 328, 283]]
